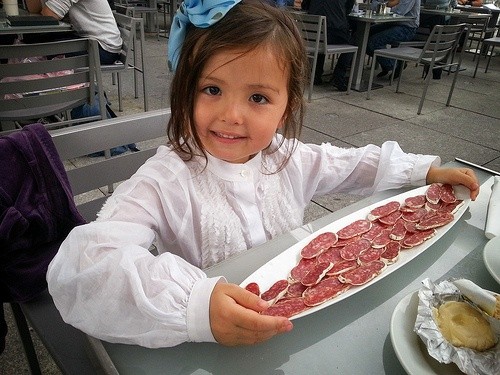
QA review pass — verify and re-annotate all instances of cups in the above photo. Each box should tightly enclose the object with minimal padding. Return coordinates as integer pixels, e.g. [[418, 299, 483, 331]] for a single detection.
[[2, 0, 18, 16], [386, 7, 391, 13]]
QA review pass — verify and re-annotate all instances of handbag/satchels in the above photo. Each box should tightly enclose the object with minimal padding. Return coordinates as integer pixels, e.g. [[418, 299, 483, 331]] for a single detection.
[[70, 90, 139, 157]]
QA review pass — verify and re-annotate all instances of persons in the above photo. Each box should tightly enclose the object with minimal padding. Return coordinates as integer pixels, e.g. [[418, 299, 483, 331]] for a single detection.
[[300, 0, 355, 89], [25, 0, 123, 65], [359, 0, 421, 81], [46, 0, 480, 349], [424, 0, 483, 79]]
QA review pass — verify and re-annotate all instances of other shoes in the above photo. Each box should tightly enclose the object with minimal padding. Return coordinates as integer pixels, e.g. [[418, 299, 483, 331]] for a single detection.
[[329, 77, 348, 91], [377, 65, 394, 78], [389, 60, 408, 79], [314, 79, 328, 86]]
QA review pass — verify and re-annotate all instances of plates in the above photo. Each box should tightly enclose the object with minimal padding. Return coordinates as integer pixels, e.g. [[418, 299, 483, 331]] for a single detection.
[[483, 235, 500, 285], [353, 12, 365, 15], [238, 182, 473, 323], [390, 286, 500, 375]]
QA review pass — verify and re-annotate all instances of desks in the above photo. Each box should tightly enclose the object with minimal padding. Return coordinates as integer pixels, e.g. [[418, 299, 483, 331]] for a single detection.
[[322, 12, 418, 93], [0, 7, 75, 43], [454, 6, 500, 57], [420, 5, 493, 74], [100, 156, 500, 375]]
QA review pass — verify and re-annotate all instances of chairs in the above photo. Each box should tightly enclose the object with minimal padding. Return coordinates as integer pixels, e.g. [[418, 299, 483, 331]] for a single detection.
[[472, 36, 500, 78], [114, 0, 160, 42], [365, 22, 473, 115], [0, 37, 114, 196], [71, 11, 148, 112], [375, 24, 432, 87], [292, 12, 359, 103], [0, 107, 171, 375]]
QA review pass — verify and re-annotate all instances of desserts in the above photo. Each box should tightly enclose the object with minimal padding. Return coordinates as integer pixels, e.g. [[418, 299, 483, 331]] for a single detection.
[[437, 301, 498, 350]]
